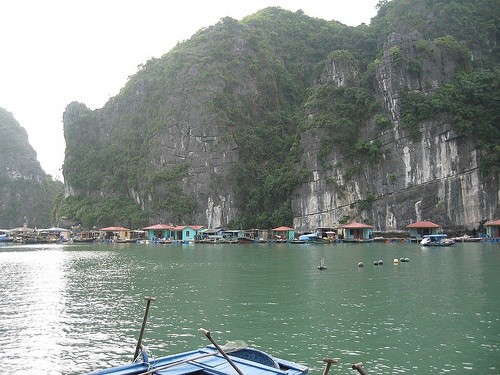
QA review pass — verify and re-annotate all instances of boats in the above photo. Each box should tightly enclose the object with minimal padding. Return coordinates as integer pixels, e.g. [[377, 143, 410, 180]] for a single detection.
[[0, 229, 97, 242], [420, 234, 456, 247], [84, 342, 310, 375], [236, 237, 254, 244], [117, 237, 190, 245], [451, 235, 483, 243]]
